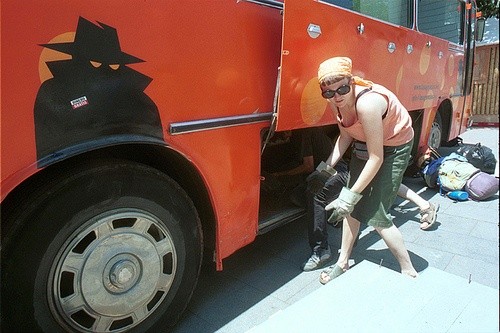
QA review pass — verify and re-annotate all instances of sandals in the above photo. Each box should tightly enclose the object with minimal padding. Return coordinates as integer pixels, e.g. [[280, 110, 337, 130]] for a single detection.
[[419, 201, 440, 229]]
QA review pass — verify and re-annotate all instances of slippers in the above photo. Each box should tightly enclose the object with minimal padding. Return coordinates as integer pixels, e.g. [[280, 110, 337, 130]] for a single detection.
[[319, 264, 344, 285]]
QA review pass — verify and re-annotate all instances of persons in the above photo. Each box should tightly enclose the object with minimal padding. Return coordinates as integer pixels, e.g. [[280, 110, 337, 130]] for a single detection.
[[304, 55, 420, 283], [264, 129, 348, 271], [312, 125, 440, 230]]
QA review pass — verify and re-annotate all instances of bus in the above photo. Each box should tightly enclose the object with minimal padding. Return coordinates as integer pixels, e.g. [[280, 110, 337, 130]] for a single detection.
[[0, 0, 486, 333]]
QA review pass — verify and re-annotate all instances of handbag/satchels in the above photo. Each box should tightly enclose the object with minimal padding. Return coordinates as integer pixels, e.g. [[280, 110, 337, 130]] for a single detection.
[[421, 137, 499, 200]]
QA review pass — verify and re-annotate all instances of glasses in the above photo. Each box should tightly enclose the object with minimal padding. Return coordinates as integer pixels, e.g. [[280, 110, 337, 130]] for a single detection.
[[322, 79, 352, 99]]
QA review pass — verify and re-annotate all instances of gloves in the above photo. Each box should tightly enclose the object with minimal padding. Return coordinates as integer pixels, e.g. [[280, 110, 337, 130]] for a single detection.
[[325, 187, 363, 227], [305, 160, 338, 198]]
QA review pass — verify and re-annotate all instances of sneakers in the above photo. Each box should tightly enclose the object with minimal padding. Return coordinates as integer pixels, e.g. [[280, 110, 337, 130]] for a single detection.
[[300, 244, 332, 271]]
